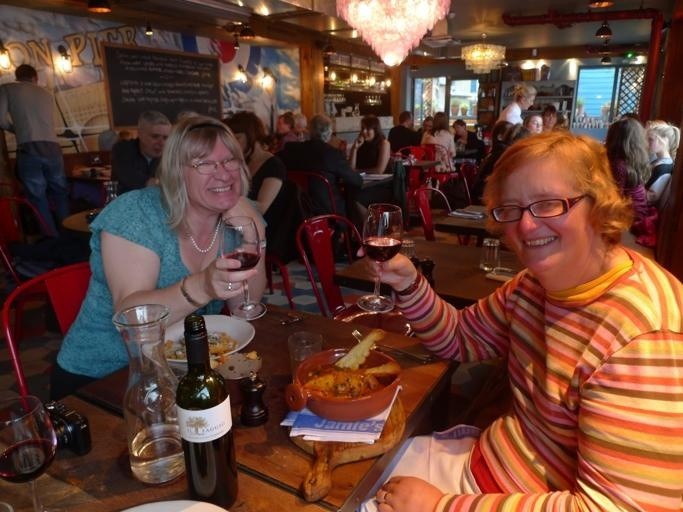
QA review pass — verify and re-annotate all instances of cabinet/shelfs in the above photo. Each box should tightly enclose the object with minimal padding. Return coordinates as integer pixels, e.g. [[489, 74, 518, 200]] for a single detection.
[[476, 81, 499, 123], [499, 79, 578, 131]]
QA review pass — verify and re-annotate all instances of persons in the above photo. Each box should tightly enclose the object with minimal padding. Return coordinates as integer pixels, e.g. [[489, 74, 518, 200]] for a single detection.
[[477, 84, 559, 196], [0, 64, 70, 241], [111, 110, 199, 198], [49, 116, 265, 404], [222, 109, 365, 277], [606, 113, 683, 250], [357, 133, 683, 512], [348, 109, 478, 220]]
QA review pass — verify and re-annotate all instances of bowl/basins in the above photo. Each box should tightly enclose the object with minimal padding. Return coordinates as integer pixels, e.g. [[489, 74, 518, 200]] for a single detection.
[[283, 345, 403, 421]]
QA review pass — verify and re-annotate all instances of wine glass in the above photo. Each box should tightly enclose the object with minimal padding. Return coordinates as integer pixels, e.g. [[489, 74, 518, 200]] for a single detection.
[[0, 395, 56, 511], [354, 203, 402, 314], [216, 215, 268, 320]]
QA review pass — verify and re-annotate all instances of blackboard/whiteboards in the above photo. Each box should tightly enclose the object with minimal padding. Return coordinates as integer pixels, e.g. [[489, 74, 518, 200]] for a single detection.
[[99, 42, 224, 132]]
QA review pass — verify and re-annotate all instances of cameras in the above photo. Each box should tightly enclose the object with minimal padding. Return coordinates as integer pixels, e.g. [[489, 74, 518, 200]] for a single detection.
[[39, 401, 91, 456]]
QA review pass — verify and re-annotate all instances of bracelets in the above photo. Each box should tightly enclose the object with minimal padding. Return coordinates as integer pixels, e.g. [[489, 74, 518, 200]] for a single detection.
[[393, 269, 422, 297], [181, 276, 206, 308], [244, 238, 265, 248]]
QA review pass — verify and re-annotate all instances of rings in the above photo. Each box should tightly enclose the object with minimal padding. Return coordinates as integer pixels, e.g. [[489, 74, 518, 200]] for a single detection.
[[384, 493, 390, 503], [228, 282, 231, 290]]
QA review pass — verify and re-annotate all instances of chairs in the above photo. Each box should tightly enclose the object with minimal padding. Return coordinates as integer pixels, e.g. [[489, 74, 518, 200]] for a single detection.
[[2, 260, 92, 420], [264, 213, 419, 339], [1, 194, 60, 347], [392, 139, 495, 236]]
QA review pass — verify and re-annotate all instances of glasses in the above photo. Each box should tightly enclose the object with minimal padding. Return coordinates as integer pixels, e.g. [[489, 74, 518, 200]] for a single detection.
[[488, 193, 590, 222], [187, 157, 241, 174]]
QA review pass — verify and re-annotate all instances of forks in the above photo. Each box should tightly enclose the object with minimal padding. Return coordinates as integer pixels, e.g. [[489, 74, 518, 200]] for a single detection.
[[350, 330, 432, 364]]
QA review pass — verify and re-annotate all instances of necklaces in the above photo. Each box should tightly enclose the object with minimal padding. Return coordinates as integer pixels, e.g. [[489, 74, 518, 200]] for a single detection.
[[185, 214, 222, 253]]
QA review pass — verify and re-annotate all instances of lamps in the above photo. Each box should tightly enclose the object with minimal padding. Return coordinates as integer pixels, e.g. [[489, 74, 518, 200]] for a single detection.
[[231, 38, 240, 51], [224, 20, 257, 42], [599, 53, 613, 66], [458, 28, 511, 78], [143, 22, 155, 37], [332, 1, 452, 70], [592, 18, 619, 42]]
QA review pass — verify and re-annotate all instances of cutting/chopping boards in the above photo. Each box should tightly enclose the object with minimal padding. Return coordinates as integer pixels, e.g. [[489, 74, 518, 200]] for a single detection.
[[287, 397, 406, 503]]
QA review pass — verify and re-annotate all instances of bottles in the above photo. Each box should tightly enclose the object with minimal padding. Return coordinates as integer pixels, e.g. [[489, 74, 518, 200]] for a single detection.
[[479, 237, 502, 272], [402, 239, 438, 289], [240, 370, 268, 426], [102, 181, 117, 205], [111, 303, 186, 488], [175, 313, 238, 510]]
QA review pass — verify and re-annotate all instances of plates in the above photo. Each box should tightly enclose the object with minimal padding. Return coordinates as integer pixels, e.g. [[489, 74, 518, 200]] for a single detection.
[[112, 499, 225, 511], [141, 312, 256, 368]]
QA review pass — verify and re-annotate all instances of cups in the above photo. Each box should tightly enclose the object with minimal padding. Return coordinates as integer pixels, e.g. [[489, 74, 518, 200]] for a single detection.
[[283, 330, 325, 384]]
